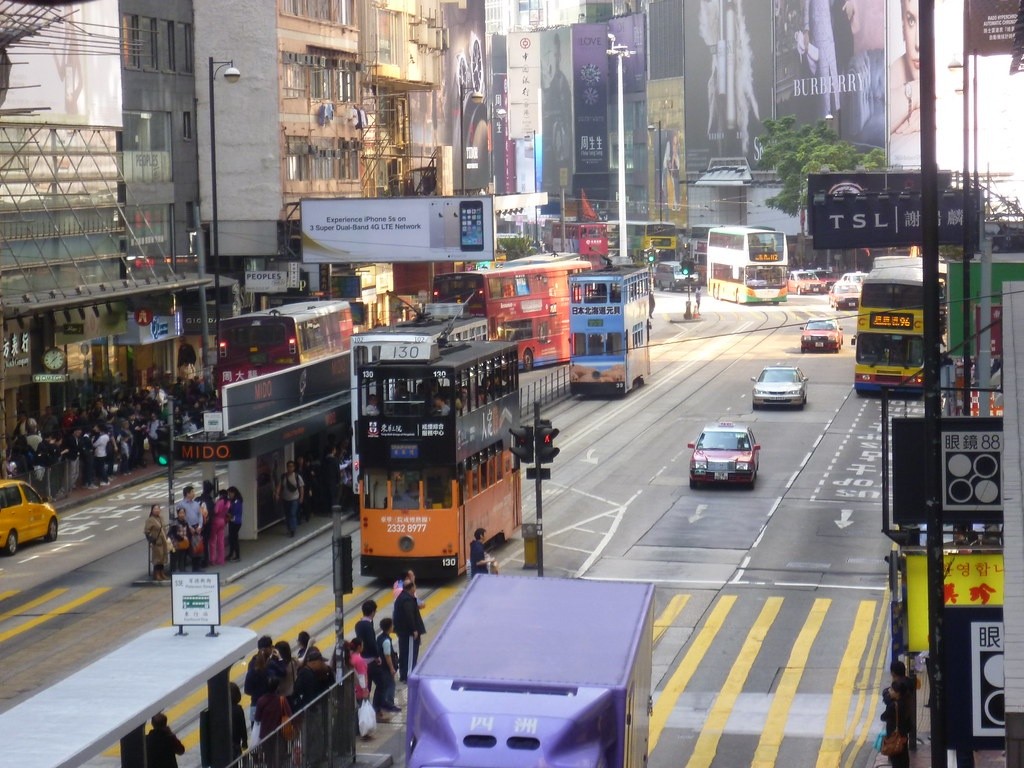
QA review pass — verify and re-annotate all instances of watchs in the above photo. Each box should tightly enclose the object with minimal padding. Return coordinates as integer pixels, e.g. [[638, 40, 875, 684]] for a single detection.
[[454, 31, 483, 101]]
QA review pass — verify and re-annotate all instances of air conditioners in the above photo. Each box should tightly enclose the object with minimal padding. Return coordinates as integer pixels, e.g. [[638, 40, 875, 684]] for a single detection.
[[391, 158, 403, 176], [293, 137, 362, 159], [280, 51, 350, 70]]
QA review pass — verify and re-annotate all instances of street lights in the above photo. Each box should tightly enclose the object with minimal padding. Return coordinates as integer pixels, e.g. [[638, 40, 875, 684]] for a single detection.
[[606, 30, 638, 261], [646, 121, 664, 222], [457, 81, 486, 273], [206, 55, 241, 318]]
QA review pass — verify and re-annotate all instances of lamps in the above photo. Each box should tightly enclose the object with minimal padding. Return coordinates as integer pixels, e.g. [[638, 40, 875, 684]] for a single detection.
[[17, 315, 24, 329], [33, 313, 41, 326], [99, 284, 105, 291], [49, 290, 56, 297], [146, 276, 152, 284], [496, 207, 524, 217], [23, 294, 30, 302], [92, 303, 99, 317], [164, 274, 170, 281], [181, 272, 187, 279], [122, 280, 129, 287], [106, 302, 112, 314], [75, 287, 83, 294], [63, 310, 71, 322], [78, 307, 85, 319]]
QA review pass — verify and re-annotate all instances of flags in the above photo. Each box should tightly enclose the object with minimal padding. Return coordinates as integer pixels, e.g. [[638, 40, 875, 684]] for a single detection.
[[582, 189, 597, 220]]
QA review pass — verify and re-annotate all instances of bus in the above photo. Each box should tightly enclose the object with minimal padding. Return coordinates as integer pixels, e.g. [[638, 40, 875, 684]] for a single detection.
[[707, 226, 789, 306], [566, 245, 651, 399], [430, 258, 596, 375], [347, 291, 490, 518], [495, 250, 580, 268], [215, 299, 354, 412], [354, 291, 523, 589], [849, 255, 947, 401], [543, 217, 776, 291]]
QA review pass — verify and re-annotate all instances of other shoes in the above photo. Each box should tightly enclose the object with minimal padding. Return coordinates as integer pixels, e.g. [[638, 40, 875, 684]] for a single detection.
[[377, 712, 394, 722], [231, 557, 240, 563], [223, 554, 232, 560], [193, 568, 206, 572], [383, 704, 400, 712], [88, 484, 98, 489], [100, 481, 109, 486]]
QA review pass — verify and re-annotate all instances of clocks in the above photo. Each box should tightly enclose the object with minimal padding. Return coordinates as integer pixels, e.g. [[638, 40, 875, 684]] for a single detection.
[[40, 346, 67, 372]]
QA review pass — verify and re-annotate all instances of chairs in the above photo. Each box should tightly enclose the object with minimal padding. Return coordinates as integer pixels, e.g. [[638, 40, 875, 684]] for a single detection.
[[827, 324, 834, 329]]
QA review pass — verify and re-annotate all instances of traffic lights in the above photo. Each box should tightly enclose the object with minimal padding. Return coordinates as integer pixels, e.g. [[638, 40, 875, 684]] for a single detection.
[[535, 418, 560, 464], [508, 424, 534, 465], [154, 424, 172, 467]]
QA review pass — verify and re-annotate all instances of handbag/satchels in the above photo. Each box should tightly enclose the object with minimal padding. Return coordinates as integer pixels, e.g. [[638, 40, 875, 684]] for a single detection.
[[358, 697, 377, 738], [143, 438, 149, 450], [190, 533, 205, 554], [119, 442, 130, 461], [146, 532, 156, 544], [286, 482, 297, 493], [249, 721, 262, 754], [278, 696, 295, 741], [882, 732, 906, 755], [34, 465, 44, 481]]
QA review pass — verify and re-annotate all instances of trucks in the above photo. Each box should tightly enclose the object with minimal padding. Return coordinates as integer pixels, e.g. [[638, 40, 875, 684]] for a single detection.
[[400, 568, 659, 768]]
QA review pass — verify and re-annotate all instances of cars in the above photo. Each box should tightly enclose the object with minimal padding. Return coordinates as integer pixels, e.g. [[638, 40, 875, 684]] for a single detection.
[[0, 479, 59, 556], [787, 268, 828, 296], [805, 266, 841, 292], [799, 315, 844, 354], [840, 270, 869, 285], [828, 281, 861, 311], [685, 420, 761, 489], [750, 365, 808, 409]]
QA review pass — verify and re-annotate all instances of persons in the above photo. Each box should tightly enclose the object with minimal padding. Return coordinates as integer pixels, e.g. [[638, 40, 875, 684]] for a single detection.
[[0, 359, 218, 504], [230, 631, 335, 768], [373, 319, 387, 329], [404, 479, 430, 509], [880, 661, 914, 768], [803, 0, 886, 151], [696, 286, 702, 315], [275, 433, 353, 537], [393, 568, 426, 684], [144, 481, 243, 581], [177, 336, 196, 369], [49, 3, 84, 115], [364, 374, 512, 417], [330, 600, 402, 756], [890, 0, 920, 136], [661, 134, 680, 204], [649, 291, 655, 319], [145, 714, 185, 768], [470, 528, 496, 580], [542, 34, 572, 195]]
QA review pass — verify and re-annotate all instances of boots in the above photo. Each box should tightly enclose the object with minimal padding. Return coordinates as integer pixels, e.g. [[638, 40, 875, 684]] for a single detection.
[[160, 569, 170, 582], [152, 569, 161, 581]]
